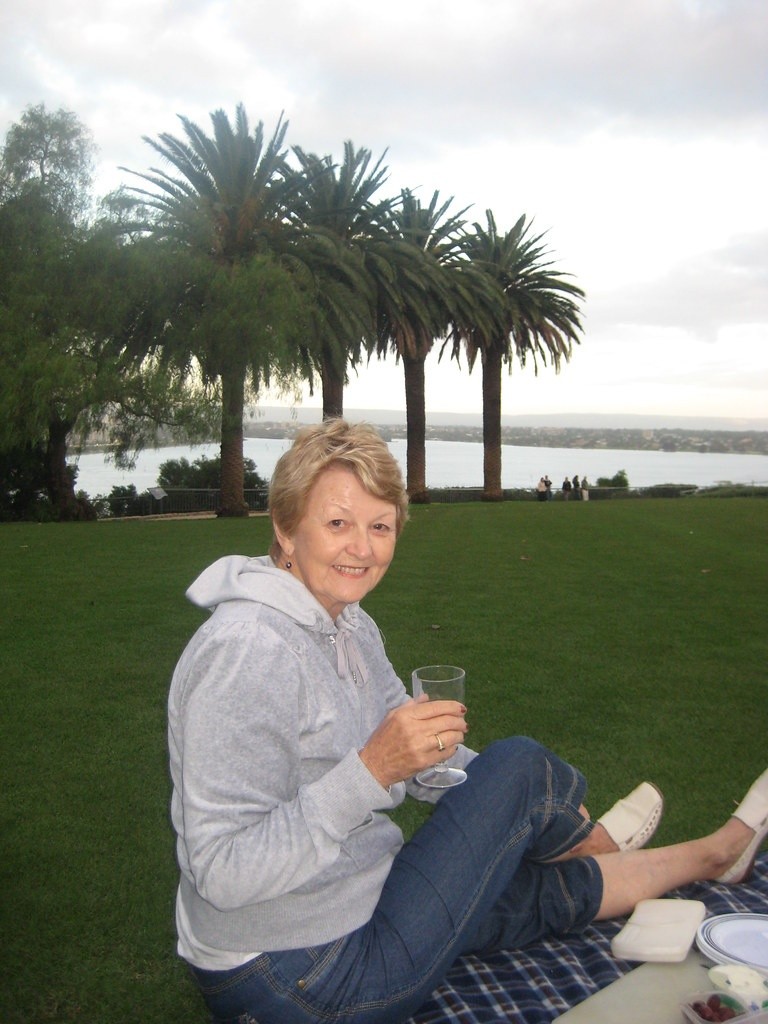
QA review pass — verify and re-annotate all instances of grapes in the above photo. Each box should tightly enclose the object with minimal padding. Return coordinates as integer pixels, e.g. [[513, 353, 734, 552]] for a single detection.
[[690, 994, 735, 1022]]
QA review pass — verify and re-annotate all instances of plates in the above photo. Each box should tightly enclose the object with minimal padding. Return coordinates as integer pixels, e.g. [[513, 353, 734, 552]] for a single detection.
[[695, 912, 768, 976]]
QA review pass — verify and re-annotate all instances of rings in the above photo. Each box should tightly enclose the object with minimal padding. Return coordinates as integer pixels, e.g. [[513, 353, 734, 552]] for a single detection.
[[435, 734, 446, 752]]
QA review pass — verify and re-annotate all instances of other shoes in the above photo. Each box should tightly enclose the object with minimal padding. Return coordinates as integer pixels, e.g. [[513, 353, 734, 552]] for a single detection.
[[597, 781, 663, 853], [714, 769, 768, 885]]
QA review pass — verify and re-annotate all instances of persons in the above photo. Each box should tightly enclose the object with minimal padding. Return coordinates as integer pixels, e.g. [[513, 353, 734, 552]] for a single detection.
[[535, 474, 590, 502], [168, 418, 767, 1024]]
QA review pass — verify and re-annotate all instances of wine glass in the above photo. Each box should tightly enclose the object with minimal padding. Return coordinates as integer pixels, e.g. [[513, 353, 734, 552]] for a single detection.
[[412, 665, 467, 788]]
